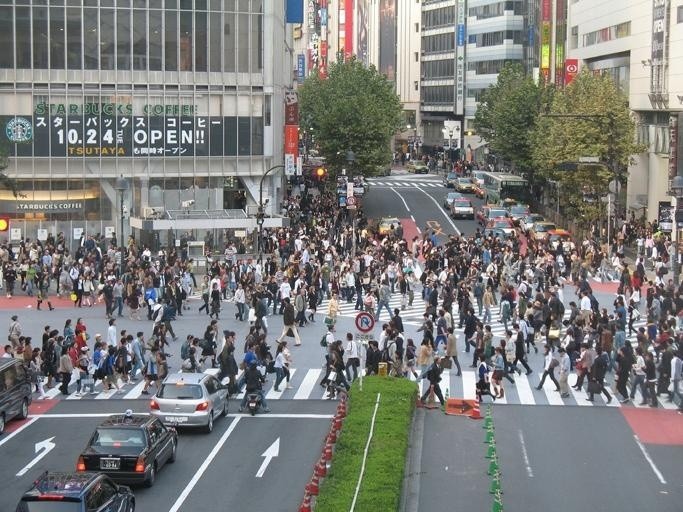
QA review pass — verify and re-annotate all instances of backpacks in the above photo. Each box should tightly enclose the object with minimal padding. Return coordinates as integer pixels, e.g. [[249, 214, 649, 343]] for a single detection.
[[380, 338, 395, 361]]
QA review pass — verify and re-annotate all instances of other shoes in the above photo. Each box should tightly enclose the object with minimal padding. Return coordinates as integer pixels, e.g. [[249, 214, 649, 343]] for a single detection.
[[5, 278, 517, 351]]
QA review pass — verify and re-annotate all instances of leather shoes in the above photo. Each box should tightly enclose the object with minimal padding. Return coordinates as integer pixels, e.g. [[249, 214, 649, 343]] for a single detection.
[[586, 397, 594, 401], [606, 397, 612, 404], [507, 368, 533, 385], [478, 399, 483, 402]]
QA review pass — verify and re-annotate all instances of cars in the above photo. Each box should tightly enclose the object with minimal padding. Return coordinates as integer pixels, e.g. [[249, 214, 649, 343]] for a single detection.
[[377, 216, 403, 238], [443, 193, 463, 210], [476, 205, 577, 253], [76, 413, 179, 485], [335, 173, 370, 196], [407, 159, 429, 174], [450, 197, 473, 219], [444, 170, 484, 198], [148, 371, 230, 433]]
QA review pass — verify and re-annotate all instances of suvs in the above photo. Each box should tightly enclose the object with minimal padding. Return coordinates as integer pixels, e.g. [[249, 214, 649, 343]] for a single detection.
[[0, 357, 33, 434], [15, 471, 135, 512]]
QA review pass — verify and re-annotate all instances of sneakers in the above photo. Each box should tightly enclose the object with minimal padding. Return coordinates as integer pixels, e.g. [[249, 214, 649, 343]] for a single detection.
[[36, 375, 160, 402], [619, 396, 658, 408], [561, 392, 569, 398]]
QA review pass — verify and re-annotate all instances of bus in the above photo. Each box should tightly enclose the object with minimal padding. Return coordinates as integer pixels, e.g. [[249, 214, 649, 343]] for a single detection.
[[483, 172, 530, 205]]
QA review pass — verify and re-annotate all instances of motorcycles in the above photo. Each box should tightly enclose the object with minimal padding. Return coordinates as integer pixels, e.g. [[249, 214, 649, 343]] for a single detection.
[[245, 375, 268, 415]]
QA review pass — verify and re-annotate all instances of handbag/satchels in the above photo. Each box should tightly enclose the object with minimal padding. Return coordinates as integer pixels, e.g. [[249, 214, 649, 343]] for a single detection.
[[267, 360, 277, 373], [575, 361, 582, 370], [587, 379, 601, 394], [671, 391, 682, 408], [549, 358, 559, 368], [507, 352, 516, 362], [8, 335, 10, 340], [406, 358, 415, 367], [524, 320, 534, 335], [548, 322, 560, 340], [440, 356, 452, 369], [475, 379, 491, 395], [327, 367, 337, 382], [354, 357, 360, 367]]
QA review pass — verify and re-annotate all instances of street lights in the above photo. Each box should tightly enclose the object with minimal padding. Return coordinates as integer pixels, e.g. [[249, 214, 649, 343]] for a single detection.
[[658, 175, 683, 287], [415, 140, 419, 159], [343, 142, 357, 223], [441, 125, 460, 171], [352, 217, 362, 257], [113, 173, 128, 272], [407, 122, 424, 150]]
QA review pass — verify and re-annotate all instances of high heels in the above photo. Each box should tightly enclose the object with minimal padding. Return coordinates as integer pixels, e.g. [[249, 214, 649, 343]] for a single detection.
[[571, 383, 582, 392]]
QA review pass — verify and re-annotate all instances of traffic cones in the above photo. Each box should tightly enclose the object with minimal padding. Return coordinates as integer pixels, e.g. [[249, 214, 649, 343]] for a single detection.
[[468, 391, 483, 419], [425, 384, 439, 409], [299, 393, 347, 511], [482, 403, 503, 511], [415, 382, 425, 408], [440, 387, 451, 413]]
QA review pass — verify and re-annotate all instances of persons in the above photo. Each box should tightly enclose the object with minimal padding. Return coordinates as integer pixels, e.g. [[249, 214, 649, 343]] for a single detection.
[[479, 162, 513, 175], [232, 191, 246, 209], [284, 175, 338, 209], [0, 207, 682, 411], [395, 151, 479, 177]]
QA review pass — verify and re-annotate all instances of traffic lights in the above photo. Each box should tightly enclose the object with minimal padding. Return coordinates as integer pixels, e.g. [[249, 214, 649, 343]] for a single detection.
[[463, 131, 474, 136], [257, 212, 264, 225], [316, 167, 325, 178]]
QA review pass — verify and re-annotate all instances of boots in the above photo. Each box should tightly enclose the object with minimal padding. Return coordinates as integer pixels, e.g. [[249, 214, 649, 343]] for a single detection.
[[493, 387, 504, 402], [533, 385, 541, 391], [554, 386, 559, 391], [527, 347, 538, 354]]
